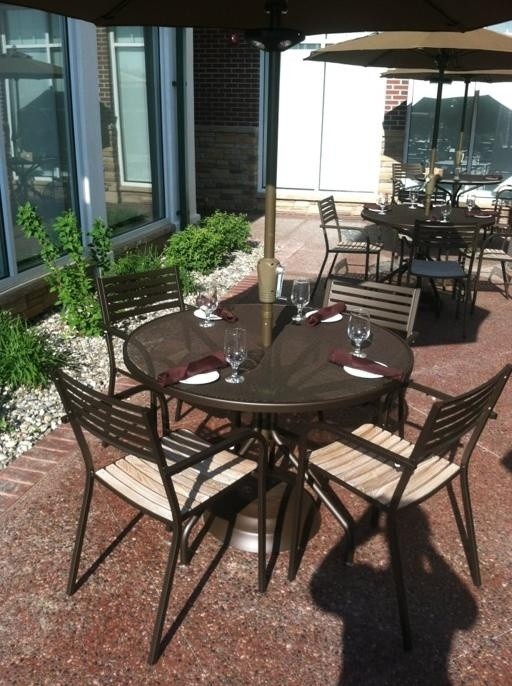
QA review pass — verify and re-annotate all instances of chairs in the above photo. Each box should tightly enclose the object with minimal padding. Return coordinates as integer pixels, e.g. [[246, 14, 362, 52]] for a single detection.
[[288, 364, 512, 655], [97, 264, 190, 449], [362, 162, 511, 339], [322, 278, 421, 344], [54, 367, 268, 666], [309, 196, 383, 305]]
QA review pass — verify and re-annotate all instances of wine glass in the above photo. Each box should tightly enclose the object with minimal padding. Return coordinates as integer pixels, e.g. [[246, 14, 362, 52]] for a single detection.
[[347, 307, 371, 359], [222, 328, 249, 384], [290, 278, 311, 322], [195, 281, 218, 328], [375, 185, 477, 223]]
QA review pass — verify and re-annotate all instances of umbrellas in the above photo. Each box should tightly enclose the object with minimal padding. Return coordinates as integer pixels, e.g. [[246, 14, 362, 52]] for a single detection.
[[300, 28, 512, 214], [0, 44, 62, 154], [0, 0, 512, 349], [379, 68, 512, 177]]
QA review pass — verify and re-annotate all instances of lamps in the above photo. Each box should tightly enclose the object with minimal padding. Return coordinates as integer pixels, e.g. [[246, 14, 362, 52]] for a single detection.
[[245, 26, 304, 49]]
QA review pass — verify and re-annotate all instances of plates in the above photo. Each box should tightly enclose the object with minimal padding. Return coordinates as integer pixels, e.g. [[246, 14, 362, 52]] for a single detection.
[[193, 309, 223, 320], [304, 309, 343, 322], [178, 370, 221, 386], [343, 362, 388, 379], [474, 214, 492, 219], [368, 208, 387, 212]]
